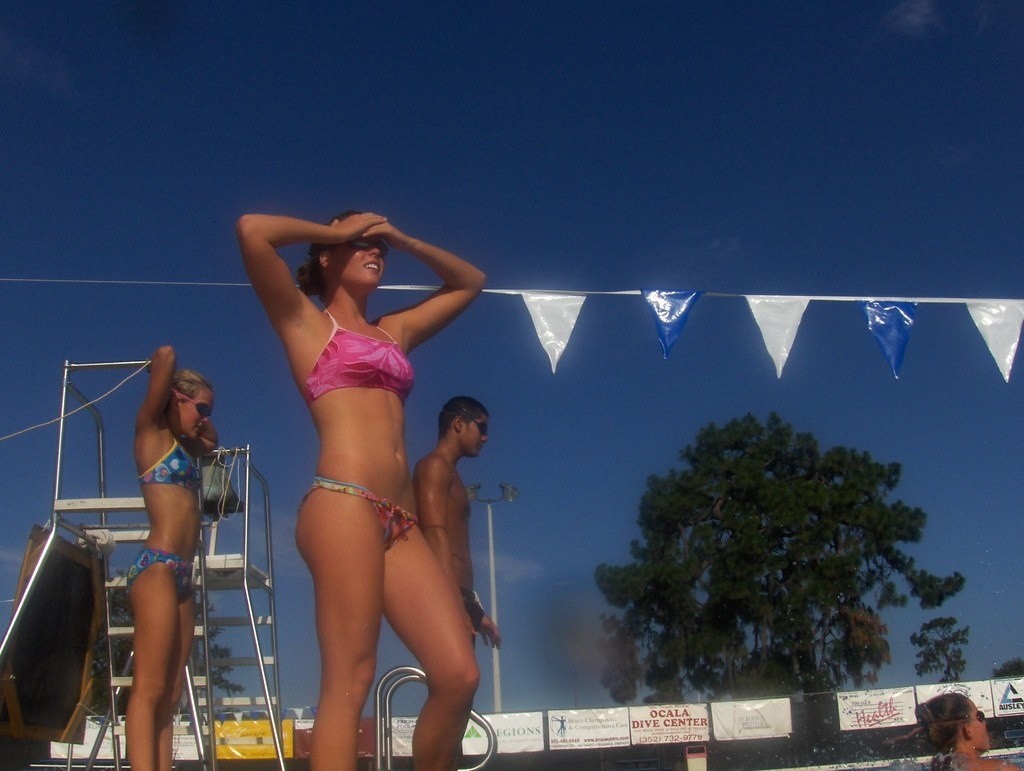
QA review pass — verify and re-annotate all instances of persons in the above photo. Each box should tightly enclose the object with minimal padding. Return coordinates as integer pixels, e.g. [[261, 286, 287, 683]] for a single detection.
[[236, 209, 486, 771], [125, 346, 219, 771], [883, 692, 1021, 771], [412, 396, 502, 771]]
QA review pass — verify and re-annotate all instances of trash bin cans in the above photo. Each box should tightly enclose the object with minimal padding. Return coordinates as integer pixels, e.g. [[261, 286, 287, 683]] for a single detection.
[[684, 745, 708, 771]]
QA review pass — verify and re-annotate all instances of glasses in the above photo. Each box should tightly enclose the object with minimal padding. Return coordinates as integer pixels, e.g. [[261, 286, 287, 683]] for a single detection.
[[184, 396, 211, 417], [465, 414, 487, 435], [977, 711, 985, 722], [348, 238, 390, 252]]
